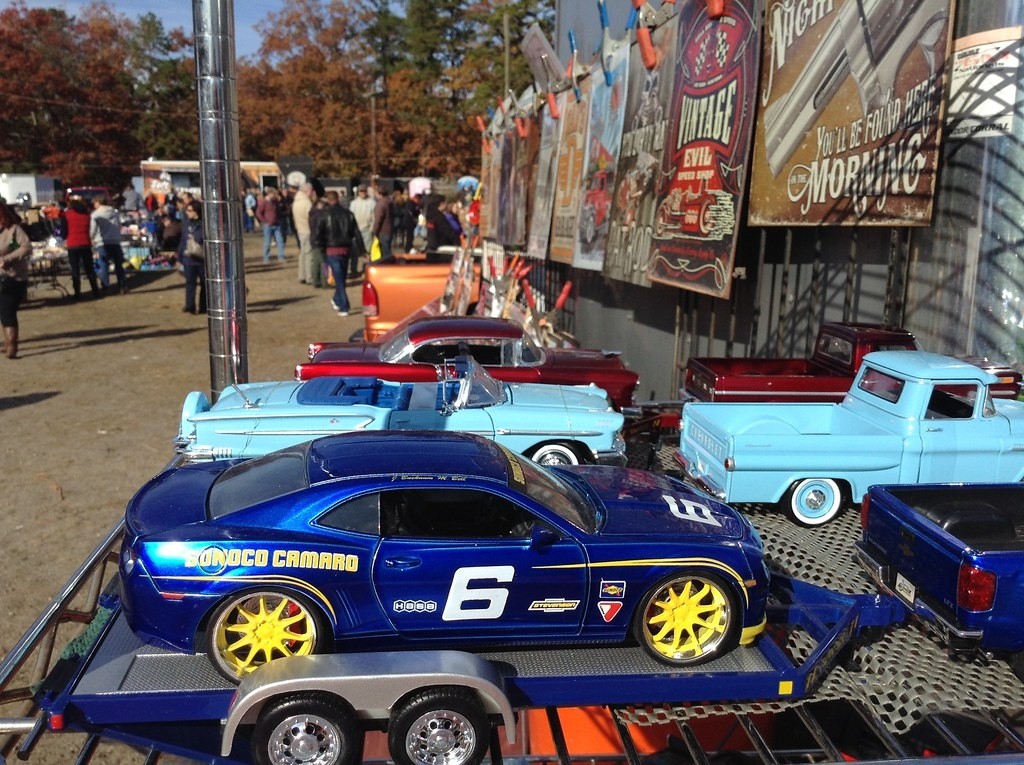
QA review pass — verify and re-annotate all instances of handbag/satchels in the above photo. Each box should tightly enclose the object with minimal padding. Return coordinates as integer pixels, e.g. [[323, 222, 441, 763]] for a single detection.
[[10, 223, 30, 262]]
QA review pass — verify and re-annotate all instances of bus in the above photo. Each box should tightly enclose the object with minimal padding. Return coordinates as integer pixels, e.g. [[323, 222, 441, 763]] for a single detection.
[[139, 159, 285, 209]]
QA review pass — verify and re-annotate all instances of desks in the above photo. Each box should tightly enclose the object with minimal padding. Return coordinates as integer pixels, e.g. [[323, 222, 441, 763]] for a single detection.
[[28, 247, 69, 300], [120, 232, 157, 269]]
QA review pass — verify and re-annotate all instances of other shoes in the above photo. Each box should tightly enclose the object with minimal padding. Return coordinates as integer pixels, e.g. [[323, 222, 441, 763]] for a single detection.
[[329, 299, 338, 309], [72, 294, 81, 299], [99, 286, 110, 294], [278, 258, 287, 263], [182, 305, 194, 313], [197, 308, 207, 314], [91, 290, 100, 294], [120, 286, 129, 293], [339, 311, 349, 314]]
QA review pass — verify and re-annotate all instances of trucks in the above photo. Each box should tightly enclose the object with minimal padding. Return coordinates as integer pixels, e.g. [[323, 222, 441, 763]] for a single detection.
[[1, 172, 62, 209]]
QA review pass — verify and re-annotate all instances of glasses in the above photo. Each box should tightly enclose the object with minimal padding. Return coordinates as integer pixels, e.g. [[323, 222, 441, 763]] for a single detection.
[[359, 190, 367, 192], [185, 209, 192, 212]]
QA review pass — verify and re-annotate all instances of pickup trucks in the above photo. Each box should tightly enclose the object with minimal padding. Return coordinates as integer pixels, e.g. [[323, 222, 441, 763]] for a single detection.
[[851, 478, 1023, 693], [670, 351, 1024, 527], [360, 249, 489, 343], [677, 319, 922, 404]]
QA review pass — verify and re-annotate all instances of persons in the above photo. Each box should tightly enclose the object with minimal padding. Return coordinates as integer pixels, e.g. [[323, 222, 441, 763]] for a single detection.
[[241, 178, 481, 255], [36, 184, 197, 252], [291, 182, 313, 284], [373, 183, 395, 259], [349, 184, 377, 278], [178, 201, 207, 314], [255, 188, 287, 264], [60, 195, 101, 299], [310, 191, 369, 316], [426, 194, 462, 252], [89, 194, 130, 295], [0, 200, 32, 359]]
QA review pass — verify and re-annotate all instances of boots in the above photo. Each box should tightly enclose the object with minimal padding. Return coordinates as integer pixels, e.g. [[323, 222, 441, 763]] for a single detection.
[[4, 327, 18, 357]]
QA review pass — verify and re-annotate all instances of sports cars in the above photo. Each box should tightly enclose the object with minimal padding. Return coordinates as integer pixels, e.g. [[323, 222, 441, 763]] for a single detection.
[[177, 350, 630, 466]]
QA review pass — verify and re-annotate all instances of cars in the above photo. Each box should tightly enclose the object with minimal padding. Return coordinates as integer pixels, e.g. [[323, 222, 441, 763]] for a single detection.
[[292, 317, 641, 411], [114, 430, 773, 683], [64, 185, 112, 209]]
